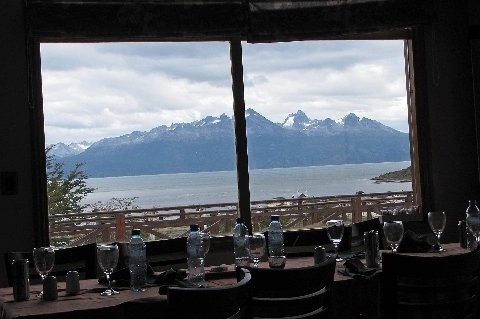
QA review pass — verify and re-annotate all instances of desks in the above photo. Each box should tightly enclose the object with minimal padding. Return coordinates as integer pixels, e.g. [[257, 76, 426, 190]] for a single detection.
[[0, 238, 480, 319]]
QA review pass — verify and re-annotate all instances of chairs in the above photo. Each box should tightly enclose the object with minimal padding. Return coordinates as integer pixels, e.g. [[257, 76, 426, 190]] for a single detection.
[[4, 215, 480, 319]]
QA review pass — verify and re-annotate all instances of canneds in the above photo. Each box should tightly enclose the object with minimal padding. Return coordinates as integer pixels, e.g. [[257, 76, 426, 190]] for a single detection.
[[458, 221, 468, 248], [314, 245, 325, 264], [66, 271, 80, 293], [12, 259, 30, 299], [43, 276, 58, 300], [364, 230, 380, 268]]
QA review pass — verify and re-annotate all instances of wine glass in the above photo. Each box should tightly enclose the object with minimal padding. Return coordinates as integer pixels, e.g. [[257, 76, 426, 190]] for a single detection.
[[33, 246, 55, 298], [97, 244, 119, 296], [249, 234, 266, 268], [384, 221, 404, 253], [326, 220, 345, 262], [428, 211, 447, 253]]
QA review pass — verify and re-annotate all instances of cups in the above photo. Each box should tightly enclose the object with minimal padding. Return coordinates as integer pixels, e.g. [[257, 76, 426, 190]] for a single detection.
[[199, 232, 210, 256]]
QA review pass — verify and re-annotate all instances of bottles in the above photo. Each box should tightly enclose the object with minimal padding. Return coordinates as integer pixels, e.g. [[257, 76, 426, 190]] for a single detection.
[[466, 199, 480, 251], [364, 230, 380, 267], [186, 224, 207, 288], [269, 215, 286, 269], [12, 258, 30, 300], [314, 245, 326, 265], [66, 270, 80, 293], [458, 221, 467, 248], [43, 276, 58, 301], [128, 228, 148, 292], [233, 218, 250, 267]]
